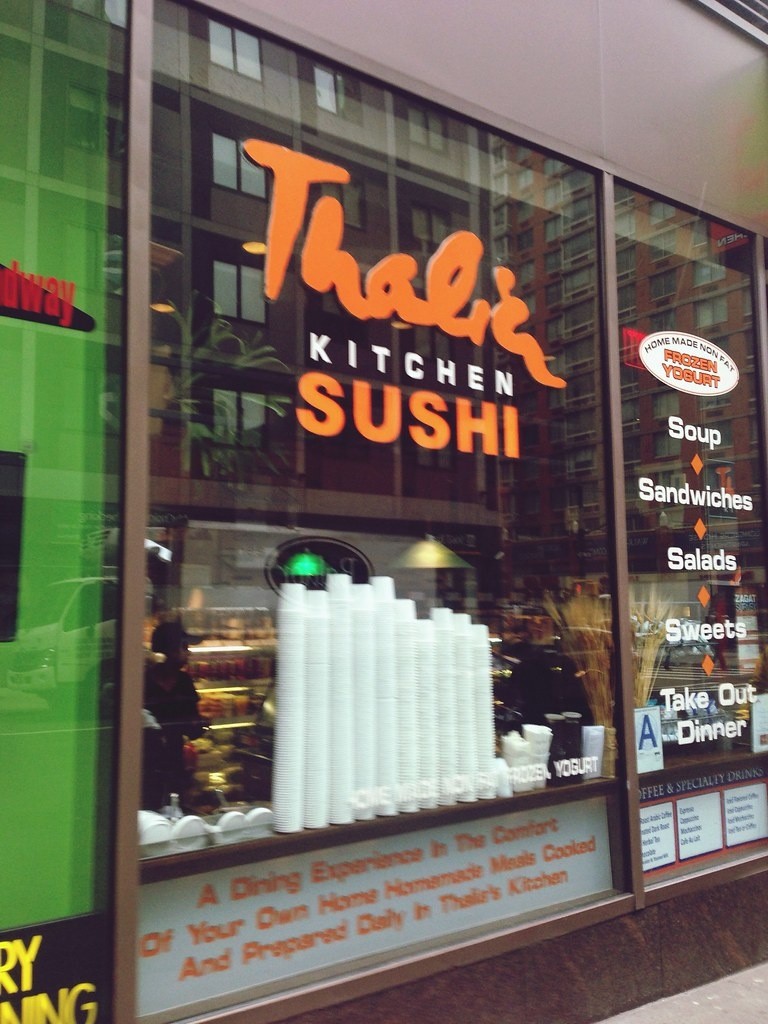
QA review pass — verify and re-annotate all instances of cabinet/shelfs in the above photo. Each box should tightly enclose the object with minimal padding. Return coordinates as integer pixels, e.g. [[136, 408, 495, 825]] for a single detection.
[[182, 646, 278, 804]]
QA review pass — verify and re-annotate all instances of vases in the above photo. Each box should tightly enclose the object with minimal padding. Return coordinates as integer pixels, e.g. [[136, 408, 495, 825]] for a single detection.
[[602, 728, 617, 779]]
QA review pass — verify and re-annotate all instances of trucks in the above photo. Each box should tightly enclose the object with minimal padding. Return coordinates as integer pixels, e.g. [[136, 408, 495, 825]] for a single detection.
[[5, 515, 467, 709]]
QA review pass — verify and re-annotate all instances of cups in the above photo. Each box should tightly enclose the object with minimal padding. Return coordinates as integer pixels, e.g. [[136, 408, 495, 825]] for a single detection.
[[271, 566, 605, 832]]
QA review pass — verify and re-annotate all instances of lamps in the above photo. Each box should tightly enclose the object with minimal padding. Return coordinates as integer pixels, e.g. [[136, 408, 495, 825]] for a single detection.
[[389, 520, 475, 569], [683, 604, 690, 617], [242, 223, 266, 254], [149, 281, 177, 313]]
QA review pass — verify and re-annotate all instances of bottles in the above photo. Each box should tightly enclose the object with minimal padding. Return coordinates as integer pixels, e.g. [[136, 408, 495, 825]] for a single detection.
[[660, 511, 668, 526]]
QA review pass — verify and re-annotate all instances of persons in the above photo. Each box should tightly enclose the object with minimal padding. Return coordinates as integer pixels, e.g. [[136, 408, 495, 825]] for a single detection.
[[142, 625, 206, 811], [704, 608, 729, 671], [664, 654, 673, 671]]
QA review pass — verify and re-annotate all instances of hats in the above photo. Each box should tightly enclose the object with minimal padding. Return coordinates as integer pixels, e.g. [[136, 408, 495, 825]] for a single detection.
[[150, 621, 203, 658]]
